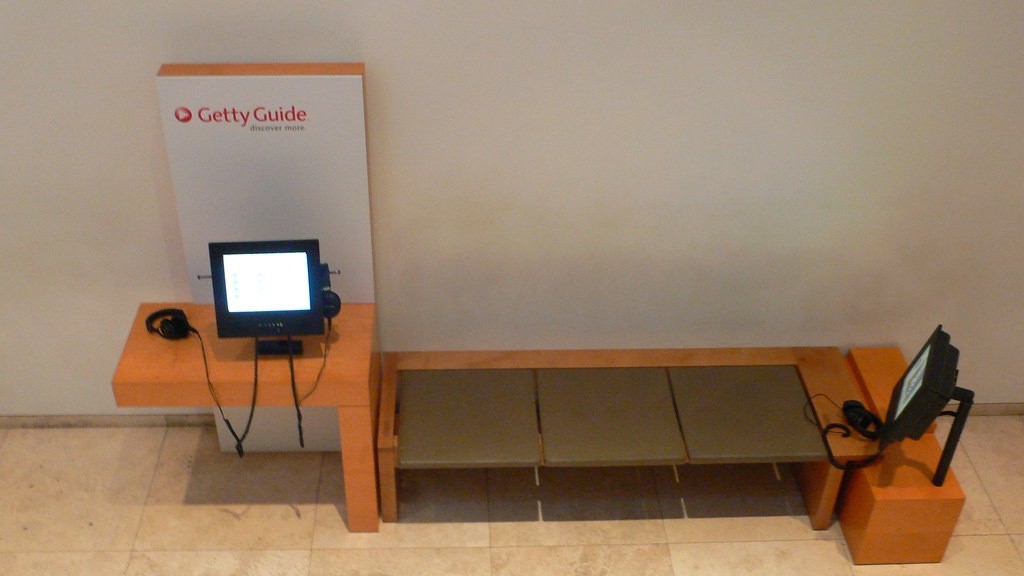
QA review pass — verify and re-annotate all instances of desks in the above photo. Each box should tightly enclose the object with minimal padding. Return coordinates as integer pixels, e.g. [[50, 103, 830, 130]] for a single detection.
[[112, 302, 385, 533]]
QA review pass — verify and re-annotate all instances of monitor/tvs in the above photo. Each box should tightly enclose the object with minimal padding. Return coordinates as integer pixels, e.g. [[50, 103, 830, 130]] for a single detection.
[[207, 239, 324, 337], [883, 324, 960, 444]]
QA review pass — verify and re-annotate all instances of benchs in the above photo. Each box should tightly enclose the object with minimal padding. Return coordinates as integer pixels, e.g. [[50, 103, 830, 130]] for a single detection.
[[376, 348, 885, 525]]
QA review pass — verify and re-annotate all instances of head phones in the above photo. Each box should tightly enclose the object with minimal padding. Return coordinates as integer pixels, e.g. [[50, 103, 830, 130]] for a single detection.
[[146, 308, 189, 339], [319, 263, 343, 318], [843, 400, 882, 439]]
